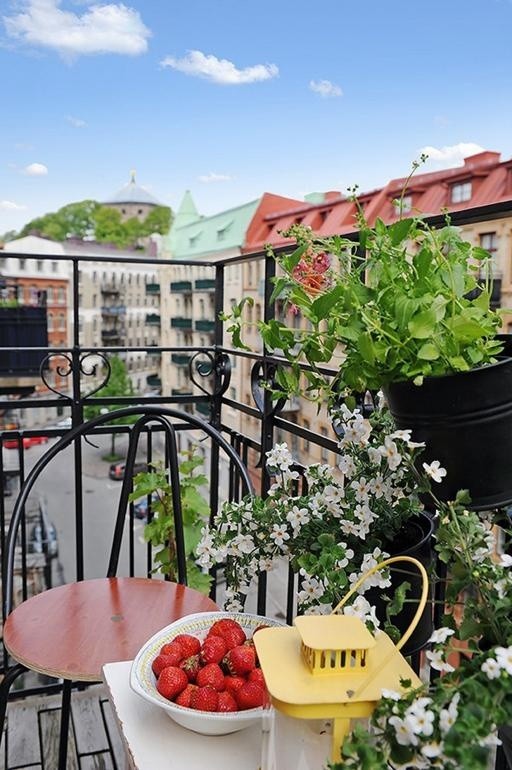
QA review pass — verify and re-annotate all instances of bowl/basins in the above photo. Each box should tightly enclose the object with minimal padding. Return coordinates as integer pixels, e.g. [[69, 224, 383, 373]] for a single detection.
[[128, 608, 300, 738]]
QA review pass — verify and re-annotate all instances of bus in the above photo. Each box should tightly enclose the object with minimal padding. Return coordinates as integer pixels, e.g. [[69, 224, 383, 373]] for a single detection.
[[0, 413, 17, 432]]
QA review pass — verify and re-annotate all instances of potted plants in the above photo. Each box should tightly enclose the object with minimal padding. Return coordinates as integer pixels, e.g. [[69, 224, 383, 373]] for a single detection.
[[219, 155, 512, 513]]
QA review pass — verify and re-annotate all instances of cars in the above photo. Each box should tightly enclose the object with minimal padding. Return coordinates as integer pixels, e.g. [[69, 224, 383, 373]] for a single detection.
[[134, 496, 161, 520], [58, 417, 72, 427], [28, 522, 58, 558], [4, 437, 49, 450], [109, 461, 157, 480], [144, 389, 160, 397]]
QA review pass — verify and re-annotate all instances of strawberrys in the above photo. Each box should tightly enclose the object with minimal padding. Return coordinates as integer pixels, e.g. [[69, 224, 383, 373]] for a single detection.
[[152, 619, 270, 712]]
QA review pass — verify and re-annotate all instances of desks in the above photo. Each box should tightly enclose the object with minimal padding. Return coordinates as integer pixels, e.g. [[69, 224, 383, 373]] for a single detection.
[[102, 660, 334, 770]]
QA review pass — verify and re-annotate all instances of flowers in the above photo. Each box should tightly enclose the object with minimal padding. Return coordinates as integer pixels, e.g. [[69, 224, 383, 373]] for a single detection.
[[196, 389, 512, 770]]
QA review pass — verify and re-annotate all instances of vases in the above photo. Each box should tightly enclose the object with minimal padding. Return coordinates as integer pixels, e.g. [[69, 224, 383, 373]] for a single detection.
[[333, 509, 434, 657]]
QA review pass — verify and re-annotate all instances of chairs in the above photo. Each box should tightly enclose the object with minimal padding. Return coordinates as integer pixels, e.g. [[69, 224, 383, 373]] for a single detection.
[[0, 406, 257, 770]]
